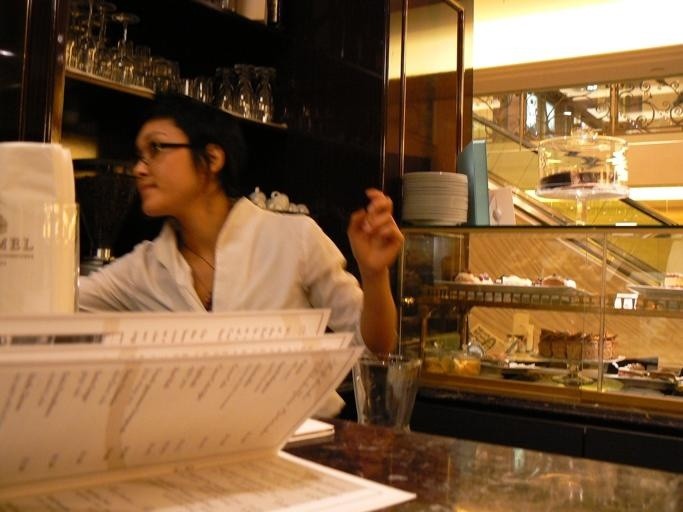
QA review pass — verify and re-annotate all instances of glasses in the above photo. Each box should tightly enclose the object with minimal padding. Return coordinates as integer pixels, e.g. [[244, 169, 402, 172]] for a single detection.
[[129, 140, 191, 165]]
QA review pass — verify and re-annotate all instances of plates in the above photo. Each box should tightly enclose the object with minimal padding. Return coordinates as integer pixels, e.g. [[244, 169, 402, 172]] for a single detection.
[[402, 172, 469, 226], [626, 284, 683, 299], [603, 373, 683, 390]]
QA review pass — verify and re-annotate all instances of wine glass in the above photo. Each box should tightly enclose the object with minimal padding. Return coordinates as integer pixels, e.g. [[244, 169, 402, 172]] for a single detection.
[[66, 0, 276, 125]]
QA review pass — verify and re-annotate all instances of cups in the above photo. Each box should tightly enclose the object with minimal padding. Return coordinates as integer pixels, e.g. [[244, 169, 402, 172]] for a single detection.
[[352, 354, 421, 433], [424, 348, 481, 377], [250, 186, 310, 214]]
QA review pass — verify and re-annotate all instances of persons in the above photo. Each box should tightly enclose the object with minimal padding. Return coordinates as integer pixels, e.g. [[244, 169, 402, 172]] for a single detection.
[[77, 91, 406, 422]]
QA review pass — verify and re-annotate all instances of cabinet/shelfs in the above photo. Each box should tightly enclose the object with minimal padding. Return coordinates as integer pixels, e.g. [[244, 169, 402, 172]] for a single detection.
[[397, 225, 683, 412], [25, 0, 391, 191]]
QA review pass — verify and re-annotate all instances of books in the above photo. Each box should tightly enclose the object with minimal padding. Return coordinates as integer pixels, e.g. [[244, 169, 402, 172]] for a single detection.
[[0, 308, 417, 512]]
